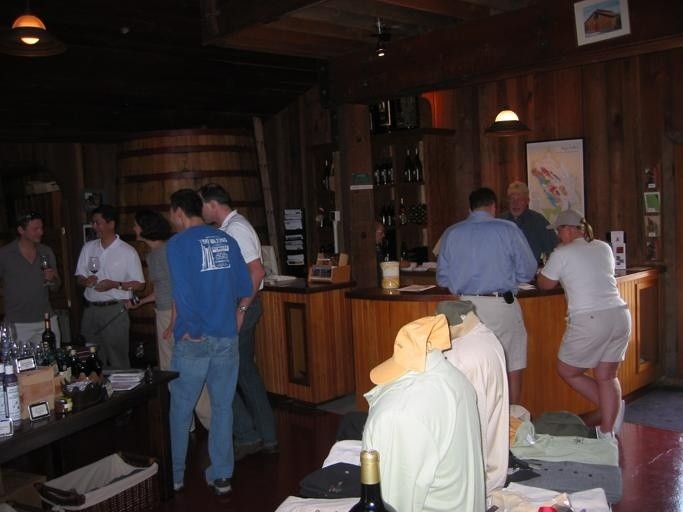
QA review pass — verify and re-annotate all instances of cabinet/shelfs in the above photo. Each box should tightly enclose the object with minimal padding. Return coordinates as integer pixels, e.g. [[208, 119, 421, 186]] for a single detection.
[[301, 86, 342, 265], [371, 126, 455, 262], [1, 167, 74, 311]]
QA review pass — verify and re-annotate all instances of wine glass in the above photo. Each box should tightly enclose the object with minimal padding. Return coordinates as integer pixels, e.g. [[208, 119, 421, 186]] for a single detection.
[[40, 256, 55, 287], [88, 257, 99, 276]]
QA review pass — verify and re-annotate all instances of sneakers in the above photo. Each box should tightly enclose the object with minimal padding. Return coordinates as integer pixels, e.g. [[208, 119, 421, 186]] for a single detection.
[[173, 482, 185, 491], [596, 426, 616, 440], [613, 399, 625, 433]]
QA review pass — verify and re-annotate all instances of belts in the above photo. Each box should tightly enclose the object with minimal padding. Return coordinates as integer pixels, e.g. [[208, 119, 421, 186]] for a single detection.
[[458, 292, 518, 297], [85, 300, 119, 306]]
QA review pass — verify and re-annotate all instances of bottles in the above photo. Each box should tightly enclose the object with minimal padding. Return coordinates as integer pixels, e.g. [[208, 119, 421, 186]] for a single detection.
[[400, 197, 407, 224], [387, 200, 393, 226], [5, 365, 22, 432], [42, 312, 55, 355], [404, 149, 413, 183], [84, 347, 102, 376], [70, 350, 80, 378], [322, 159, 330, 188], [413, 148, 423, 183], [62, 345, 73, 371], [380, 201, 387, 226], [0, 363, 6, 421], [347, 450, 397, 512]]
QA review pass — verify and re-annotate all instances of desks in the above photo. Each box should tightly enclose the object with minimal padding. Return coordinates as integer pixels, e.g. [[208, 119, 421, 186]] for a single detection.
[[254, 278, 359, 405], [0, 371, 179, 501]]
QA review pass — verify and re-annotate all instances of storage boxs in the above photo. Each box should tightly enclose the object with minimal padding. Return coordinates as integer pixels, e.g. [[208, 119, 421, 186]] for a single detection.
[[17, 367, 55, 419]]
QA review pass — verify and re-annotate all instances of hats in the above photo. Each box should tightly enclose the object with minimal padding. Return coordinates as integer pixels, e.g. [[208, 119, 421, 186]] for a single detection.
[[546, 209, 586, 228], [507, 181, 528, 197], [368, 314, 452, 388], [435, 298, 476, 326]]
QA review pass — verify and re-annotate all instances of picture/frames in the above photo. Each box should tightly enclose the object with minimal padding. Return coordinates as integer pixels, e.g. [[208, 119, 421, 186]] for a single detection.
[[524, 137, 587, 231], [574, 0, 631, 46]]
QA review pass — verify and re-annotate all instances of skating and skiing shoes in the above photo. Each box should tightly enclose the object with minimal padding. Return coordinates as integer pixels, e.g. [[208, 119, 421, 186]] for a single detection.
[[204, 467, 232, 497]]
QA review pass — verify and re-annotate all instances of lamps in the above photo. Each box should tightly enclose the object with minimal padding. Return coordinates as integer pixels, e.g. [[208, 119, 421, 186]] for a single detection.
[[373, 17, 387, 57], [484, 104, 532, 138], [0, 0, 66, 58]]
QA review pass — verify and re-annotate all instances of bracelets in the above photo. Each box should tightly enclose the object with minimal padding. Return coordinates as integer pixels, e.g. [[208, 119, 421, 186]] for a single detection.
[[118, 282, 122, 290], [238, 304, 248, 313]]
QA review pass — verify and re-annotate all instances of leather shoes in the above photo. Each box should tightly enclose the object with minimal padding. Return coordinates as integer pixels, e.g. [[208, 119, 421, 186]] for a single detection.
[[264, 440, 278, 455], [232, 438, 264, 463]]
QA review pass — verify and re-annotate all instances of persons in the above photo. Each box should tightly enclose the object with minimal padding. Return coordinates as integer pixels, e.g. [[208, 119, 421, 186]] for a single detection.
[[495, 180, 560, 264], [197, 182, 280, 461], [165, 188, 253, 496], [74, 204, 146, 428], [536, 208, 633, 440], [0, 210, 62, 349], [124, 209, 211, 432], [364, 222, 385, 266], [436, 187, 538, 404], [359, 294, 511, 512]]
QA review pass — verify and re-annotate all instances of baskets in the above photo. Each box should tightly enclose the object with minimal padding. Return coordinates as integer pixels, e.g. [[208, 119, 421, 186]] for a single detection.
[[34, 448, 163, 512]]
